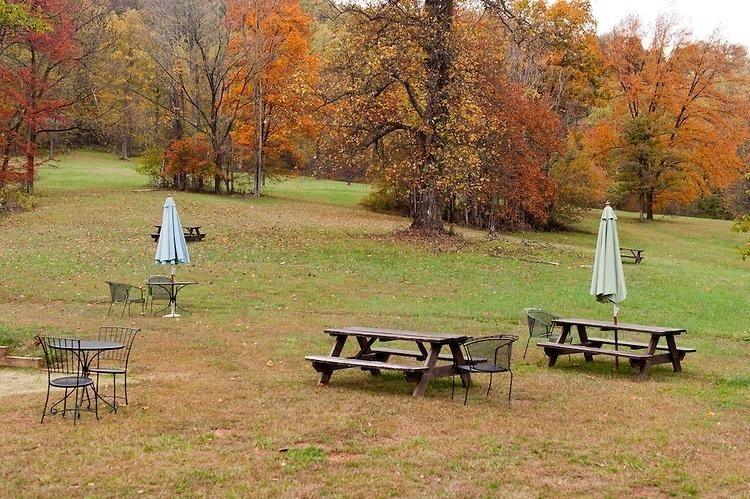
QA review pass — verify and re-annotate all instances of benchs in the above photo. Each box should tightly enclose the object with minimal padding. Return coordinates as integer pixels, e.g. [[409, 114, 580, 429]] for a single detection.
[[305, 356, 429, 372], [149, 233, 200, 243], [536, 342, 653, 359], [585, 337, 696, 352], [182, 233, 206, 238], [621, 255, 644, 259], [371, 346, 488, 363]]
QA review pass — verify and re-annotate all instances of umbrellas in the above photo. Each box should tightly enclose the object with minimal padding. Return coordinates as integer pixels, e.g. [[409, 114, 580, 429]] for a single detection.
[[590, 200, 628, 370], [154, 190, 191, 313]]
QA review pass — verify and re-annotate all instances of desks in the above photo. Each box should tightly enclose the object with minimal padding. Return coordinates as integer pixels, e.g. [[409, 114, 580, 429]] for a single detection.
[[154, 224, 201, 236], [147, 281, 198, 317], [547, 318, 686, 381], [49, 340, 124, 418], [316, 326, 472, 400], [618, 248, 644, 265]]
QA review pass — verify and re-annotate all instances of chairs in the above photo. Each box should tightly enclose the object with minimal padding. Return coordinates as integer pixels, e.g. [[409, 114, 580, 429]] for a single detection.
[[523, 308, 573, 363], [40, 336, 100, 426], [105, 281, 145, 319], [452, 335, 519, 405], [146, 275, 174, 314], [80, 326, 142, 410]]
